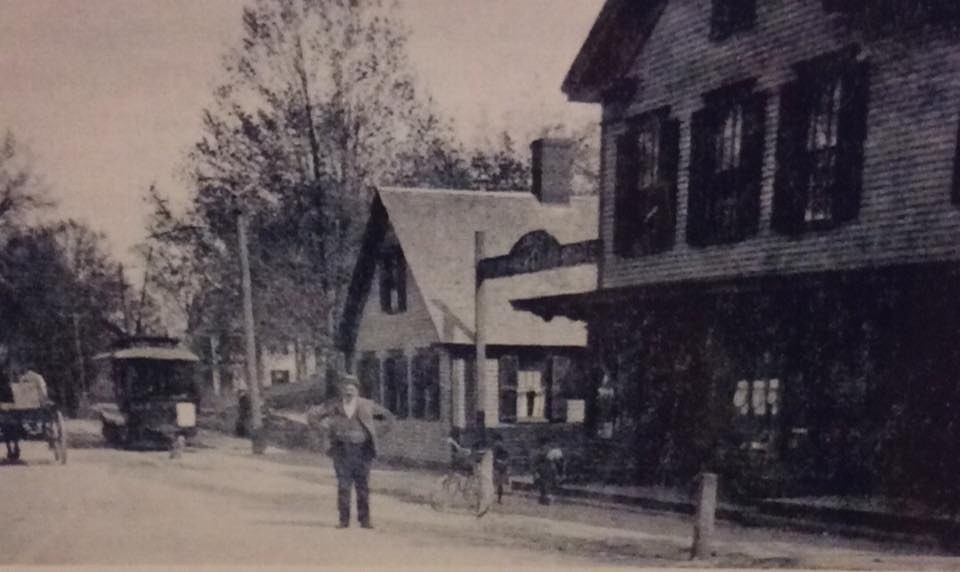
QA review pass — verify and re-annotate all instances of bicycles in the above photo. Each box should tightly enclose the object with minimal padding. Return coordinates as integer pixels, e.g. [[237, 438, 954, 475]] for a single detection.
[[428, 435, 497, 517]]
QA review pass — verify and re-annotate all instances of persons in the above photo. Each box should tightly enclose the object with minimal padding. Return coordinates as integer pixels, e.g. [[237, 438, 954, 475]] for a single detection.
[[308, 373, 394, 529], [449, 425, 467, 473], [236, 389, 253, 438], [526, 390, 544, 416], [492, 434, 509, 505], [0, 343, 48, 409], [532, 438, 562, 506]]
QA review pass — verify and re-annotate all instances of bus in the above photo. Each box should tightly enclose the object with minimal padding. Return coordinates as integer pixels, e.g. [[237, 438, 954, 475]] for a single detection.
[[83, 321, 202, 453]]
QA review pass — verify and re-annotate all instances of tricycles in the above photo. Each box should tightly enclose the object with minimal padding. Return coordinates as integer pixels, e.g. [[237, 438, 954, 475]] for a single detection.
[[0, 398, 68, 465]]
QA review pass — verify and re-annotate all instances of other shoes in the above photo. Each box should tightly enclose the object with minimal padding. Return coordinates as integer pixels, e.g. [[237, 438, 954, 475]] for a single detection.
[[336, 521, 349, 528], [360, 520, 373, 529]]
